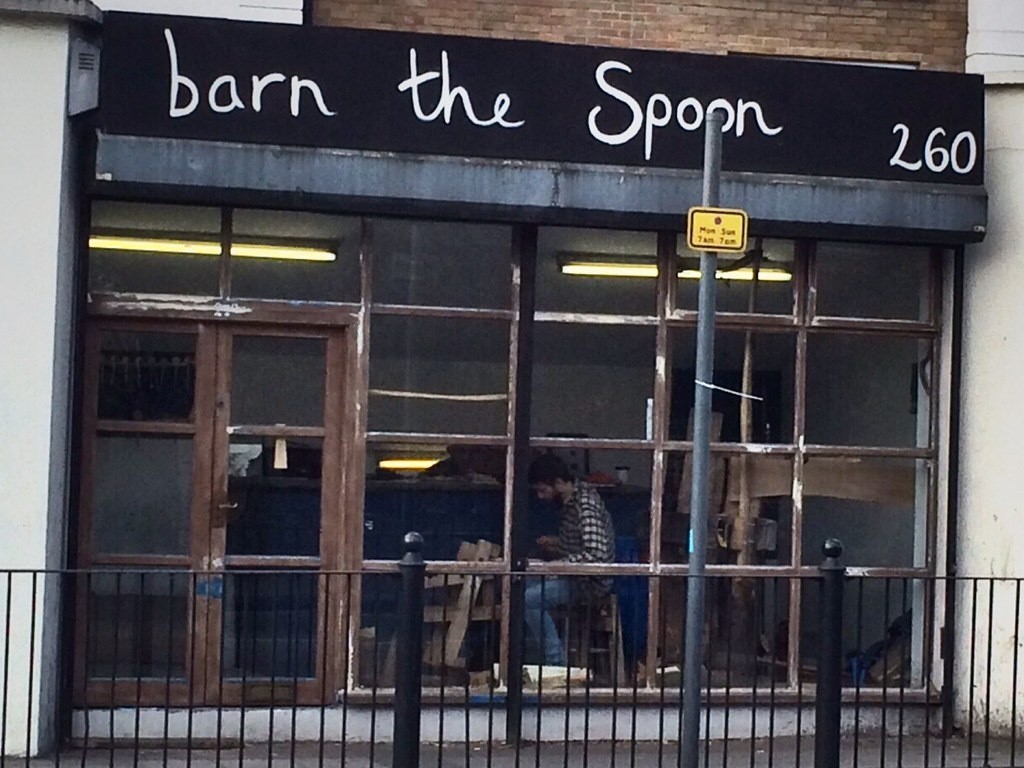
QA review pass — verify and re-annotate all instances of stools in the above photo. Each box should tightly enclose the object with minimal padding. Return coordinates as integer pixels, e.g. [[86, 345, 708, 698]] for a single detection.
[[556, 581, 627, 688]]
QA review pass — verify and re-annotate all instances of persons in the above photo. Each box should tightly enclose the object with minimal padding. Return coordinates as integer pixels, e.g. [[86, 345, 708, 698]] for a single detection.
[[523, 455, 616, 669]]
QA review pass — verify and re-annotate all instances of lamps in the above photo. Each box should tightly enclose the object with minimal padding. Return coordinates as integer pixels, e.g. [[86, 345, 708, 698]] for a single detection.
[[72, 232, 341, 259], [556, 253, 795, 281]]
